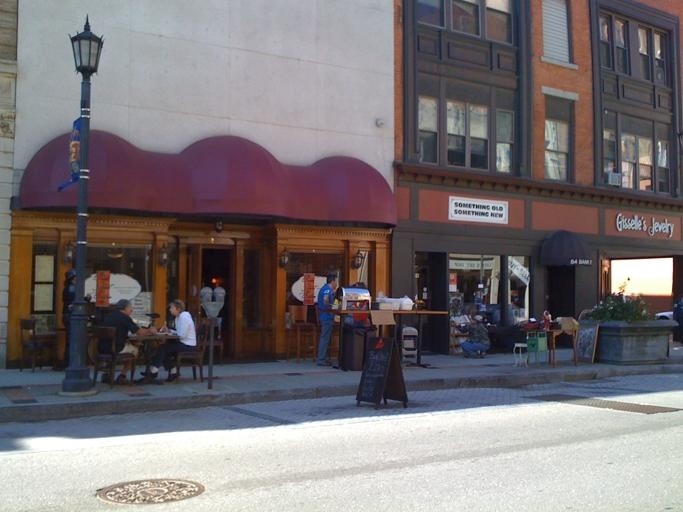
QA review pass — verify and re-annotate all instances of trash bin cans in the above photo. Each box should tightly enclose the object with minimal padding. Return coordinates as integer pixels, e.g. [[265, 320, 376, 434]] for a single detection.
[[342, 323, 377, 371]]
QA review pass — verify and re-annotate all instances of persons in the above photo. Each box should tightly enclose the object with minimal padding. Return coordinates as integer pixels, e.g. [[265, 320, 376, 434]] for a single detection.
[[450, 314, 491, 359], [62, 270, 91, 368], [139, 299, 196, 382], [672, 293, 682, 352], [315, 274, 338, 366], [96, 298, 156, 383]]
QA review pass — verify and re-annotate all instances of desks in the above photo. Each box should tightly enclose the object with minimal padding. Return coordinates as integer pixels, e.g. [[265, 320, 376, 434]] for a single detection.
[[319, 308, 449, 372]]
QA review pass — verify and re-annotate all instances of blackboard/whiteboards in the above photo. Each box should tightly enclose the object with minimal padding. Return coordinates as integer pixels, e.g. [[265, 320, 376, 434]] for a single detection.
[[572, 320, 599, 363], [364, 336, 394, 403]]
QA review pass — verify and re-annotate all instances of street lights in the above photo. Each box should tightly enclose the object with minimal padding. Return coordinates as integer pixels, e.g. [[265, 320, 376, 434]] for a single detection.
[[58, 12, 105, 399]]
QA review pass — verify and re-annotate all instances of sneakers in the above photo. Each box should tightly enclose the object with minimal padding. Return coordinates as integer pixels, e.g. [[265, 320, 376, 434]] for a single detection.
[[139, 369, 181, 382], [101, 373, 130, 385], [316, 359, 333, 367]]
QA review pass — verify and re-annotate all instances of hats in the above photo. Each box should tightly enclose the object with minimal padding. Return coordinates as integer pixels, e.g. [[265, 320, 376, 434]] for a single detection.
[[473, 314, 484, 322]]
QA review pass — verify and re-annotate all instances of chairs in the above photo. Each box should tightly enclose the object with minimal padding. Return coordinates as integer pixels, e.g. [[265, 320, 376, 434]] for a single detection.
[[91, 326, 135, 391], [285, 303, 339, 364], [171, 317, 210, 383], [17, 316, 54, 372]]
